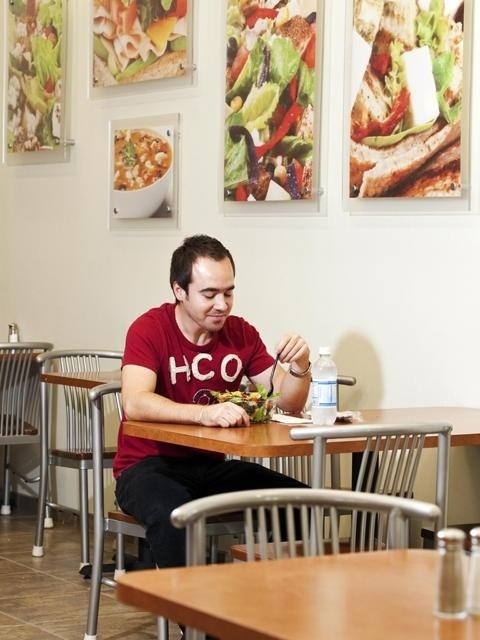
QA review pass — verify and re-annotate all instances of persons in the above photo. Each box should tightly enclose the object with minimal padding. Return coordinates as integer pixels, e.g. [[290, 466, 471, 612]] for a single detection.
[[111, 234, 315, 574]]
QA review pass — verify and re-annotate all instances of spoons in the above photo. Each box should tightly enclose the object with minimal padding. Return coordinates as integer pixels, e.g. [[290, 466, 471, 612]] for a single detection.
[[266, 353, 280, 399]]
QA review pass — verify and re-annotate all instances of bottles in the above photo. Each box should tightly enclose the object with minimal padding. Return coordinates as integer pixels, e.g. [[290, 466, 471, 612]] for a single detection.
[[7, 322, 19, 344], [465, 527, 480, 620], [308, 342, 338, 428], [430, 525, 469, 622]]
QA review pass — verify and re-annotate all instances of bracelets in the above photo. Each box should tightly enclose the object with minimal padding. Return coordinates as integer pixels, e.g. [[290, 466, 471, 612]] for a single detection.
[[289, 361, 312, 378]]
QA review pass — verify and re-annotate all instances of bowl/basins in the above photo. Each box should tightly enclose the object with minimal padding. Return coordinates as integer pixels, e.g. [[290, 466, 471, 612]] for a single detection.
[[216, 396, 279, 424], [112, 127, 175, 218]]
